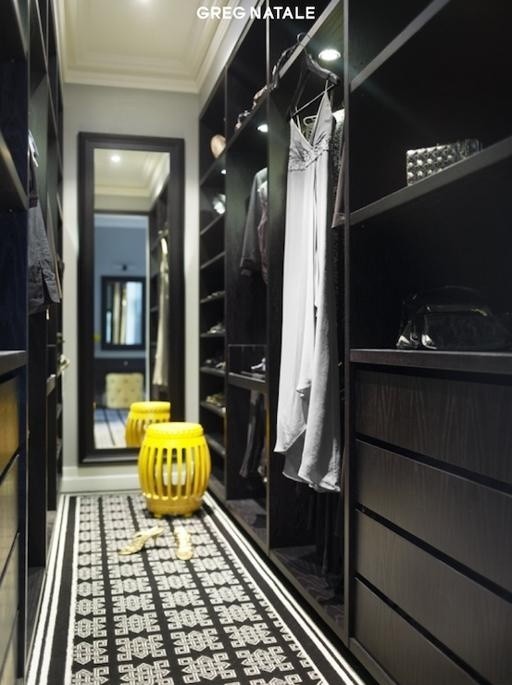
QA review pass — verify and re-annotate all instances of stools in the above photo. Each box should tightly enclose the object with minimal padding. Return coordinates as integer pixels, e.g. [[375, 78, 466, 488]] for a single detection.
[[139, 421, 211, 523], [125, 399, 171, 451], [103, 372, 144, 411]]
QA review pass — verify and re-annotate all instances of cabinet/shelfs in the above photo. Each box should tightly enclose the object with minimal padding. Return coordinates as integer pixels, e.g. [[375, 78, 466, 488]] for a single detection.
[[0, 0, 65, 685], [197, 0, 512, 684]]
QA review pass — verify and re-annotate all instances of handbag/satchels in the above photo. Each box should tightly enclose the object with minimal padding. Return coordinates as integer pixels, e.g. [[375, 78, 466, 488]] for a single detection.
[[396, 284, 509, 349], [405, 137, 485, 185]]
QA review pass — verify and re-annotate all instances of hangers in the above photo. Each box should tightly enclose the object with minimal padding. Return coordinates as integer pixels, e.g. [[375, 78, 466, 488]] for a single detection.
[[282, 30, 340, 120]]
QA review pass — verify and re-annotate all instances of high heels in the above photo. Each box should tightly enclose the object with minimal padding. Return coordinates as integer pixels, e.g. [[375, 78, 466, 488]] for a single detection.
[[117, 527, 163, 555]]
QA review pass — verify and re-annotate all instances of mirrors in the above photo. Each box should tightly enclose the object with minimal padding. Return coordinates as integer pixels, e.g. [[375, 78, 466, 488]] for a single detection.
[[73, 127, 186, 468], [99, 274, 145, 350]]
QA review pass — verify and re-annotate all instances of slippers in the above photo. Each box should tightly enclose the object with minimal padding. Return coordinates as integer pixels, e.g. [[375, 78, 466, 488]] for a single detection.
[[174, 526, 193, 561]]
